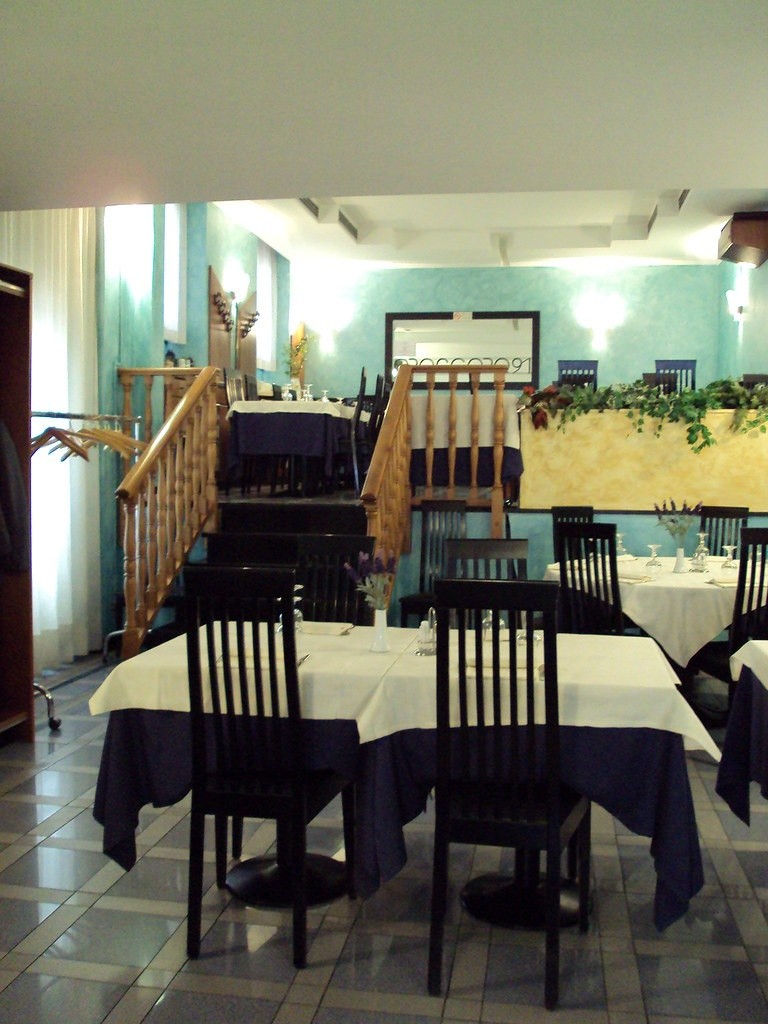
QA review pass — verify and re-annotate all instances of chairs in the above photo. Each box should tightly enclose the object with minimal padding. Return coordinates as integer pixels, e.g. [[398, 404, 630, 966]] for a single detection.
[[179, 499, 768, 1010], [221, 366, 393, 501], [551, 358, 700, 395]]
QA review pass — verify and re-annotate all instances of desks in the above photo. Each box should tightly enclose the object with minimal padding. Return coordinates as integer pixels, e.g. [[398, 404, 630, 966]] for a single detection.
[[541, 555, 768, 670], [354, 627, 722, 934], [88, 621, 420, 910], [714, 640, 768, 827], [410, 392, 524, 502], [224, 400, 381, 497]]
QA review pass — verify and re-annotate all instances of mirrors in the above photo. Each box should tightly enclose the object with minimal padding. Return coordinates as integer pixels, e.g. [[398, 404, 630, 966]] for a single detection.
[[384, 309, 541, 390]]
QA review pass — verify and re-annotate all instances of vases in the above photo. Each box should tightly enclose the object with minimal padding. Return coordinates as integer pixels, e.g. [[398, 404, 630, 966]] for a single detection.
[[370, 610, 392, 651], [672, 549, 689, 573]]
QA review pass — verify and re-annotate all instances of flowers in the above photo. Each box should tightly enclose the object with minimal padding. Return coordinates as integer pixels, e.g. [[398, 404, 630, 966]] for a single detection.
[[343, 547, 398, 609], [654, 498, 703, 548]]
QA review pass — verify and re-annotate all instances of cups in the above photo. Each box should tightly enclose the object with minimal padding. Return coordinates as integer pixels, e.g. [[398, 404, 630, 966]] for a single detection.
[[483, 610, 505, 642], [516, 631, 541, 665]]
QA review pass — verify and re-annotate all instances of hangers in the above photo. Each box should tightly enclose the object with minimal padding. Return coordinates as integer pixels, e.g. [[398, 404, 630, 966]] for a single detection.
[[30, 412, 150, 462]]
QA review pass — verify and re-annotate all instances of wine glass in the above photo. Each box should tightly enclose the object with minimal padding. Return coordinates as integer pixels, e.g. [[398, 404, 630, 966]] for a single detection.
[[276, 583, 307, 655], [696, 533, 710, 559], [644, 543, 664, 579], [721, 545, 738, 579], [615, 532, 626, 562], [281, 382, 343, 404]]
